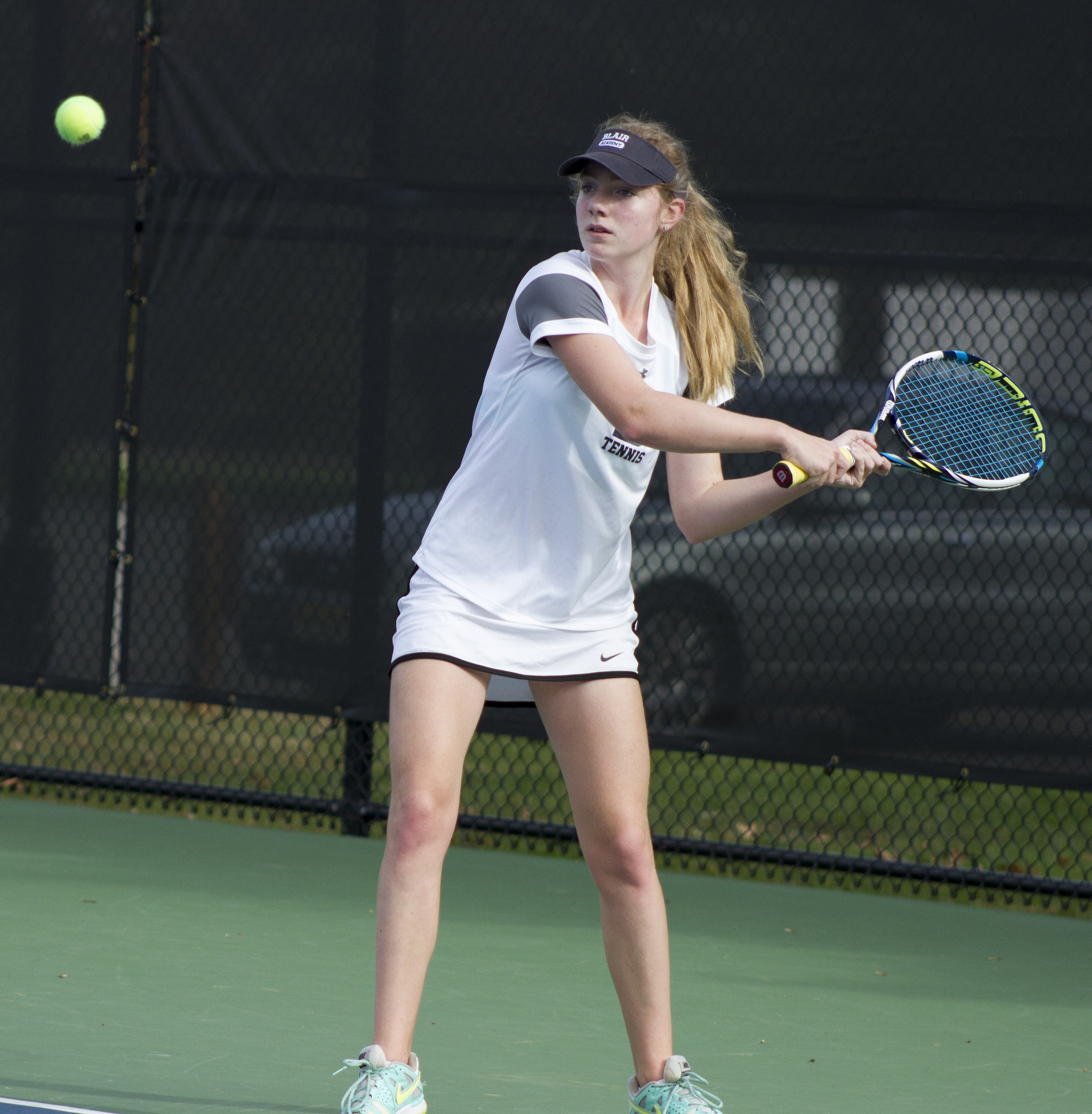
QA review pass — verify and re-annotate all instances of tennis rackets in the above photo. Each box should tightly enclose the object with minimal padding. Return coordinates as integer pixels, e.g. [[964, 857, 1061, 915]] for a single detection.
[[773, 346, 1049, 490]]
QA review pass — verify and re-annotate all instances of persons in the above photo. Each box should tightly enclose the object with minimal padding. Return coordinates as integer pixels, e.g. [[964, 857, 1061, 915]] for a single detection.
[[334, 113, 894, 1113]]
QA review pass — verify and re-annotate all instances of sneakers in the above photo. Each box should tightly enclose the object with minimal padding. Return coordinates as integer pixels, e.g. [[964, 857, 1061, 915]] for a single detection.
[[340, 1045, 427, 1114], [627, 1055, 723, 1114]]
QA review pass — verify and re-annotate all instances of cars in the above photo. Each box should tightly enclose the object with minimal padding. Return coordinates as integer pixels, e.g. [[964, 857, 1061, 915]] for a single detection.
[[248, 371, 1091, 753]]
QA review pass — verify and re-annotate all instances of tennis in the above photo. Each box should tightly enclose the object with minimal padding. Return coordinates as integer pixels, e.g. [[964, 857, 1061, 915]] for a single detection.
[[54, 92, 106, 147]]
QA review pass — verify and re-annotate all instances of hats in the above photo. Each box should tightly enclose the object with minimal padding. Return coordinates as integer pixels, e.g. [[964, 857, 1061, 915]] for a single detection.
[[557, 128, 688, 200]]
